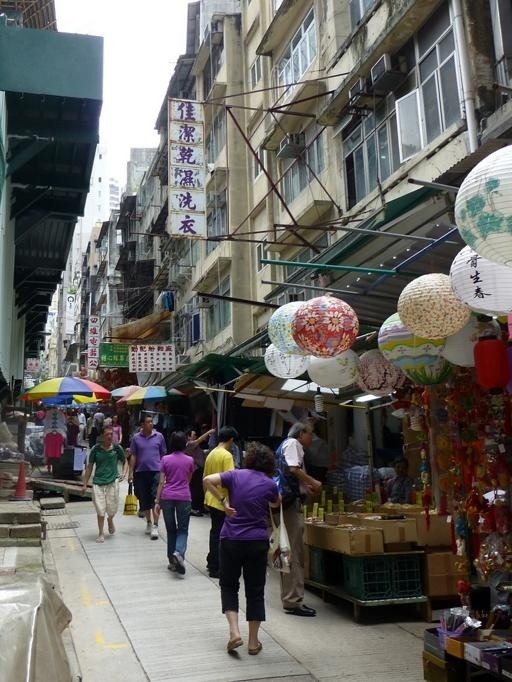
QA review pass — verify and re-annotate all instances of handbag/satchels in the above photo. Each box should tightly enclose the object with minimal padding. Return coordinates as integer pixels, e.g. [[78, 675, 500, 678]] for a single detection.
[[121, 480, 141, 516], [268, 498, 292, 575]]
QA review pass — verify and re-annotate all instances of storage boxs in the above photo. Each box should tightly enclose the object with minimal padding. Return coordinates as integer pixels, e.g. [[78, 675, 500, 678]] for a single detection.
[[415, 626, 512, 682], [303, 497, 458, 610]]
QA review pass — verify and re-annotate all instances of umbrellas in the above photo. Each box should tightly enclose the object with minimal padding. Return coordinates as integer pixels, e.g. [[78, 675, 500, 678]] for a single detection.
[[23, 375, 113, 448], [17, 384, 188, 419]]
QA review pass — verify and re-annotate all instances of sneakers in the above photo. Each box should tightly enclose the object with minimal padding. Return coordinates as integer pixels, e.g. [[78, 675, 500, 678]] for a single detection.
[[145, 521, 152, 534], [167, 562, 177, 571], [168, 550, 185, 575], [150, 524, 159, 540]]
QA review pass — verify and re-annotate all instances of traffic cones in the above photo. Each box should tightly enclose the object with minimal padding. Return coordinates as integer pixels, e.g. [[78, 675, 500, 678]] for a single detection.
[[8, 461, 32, 501]]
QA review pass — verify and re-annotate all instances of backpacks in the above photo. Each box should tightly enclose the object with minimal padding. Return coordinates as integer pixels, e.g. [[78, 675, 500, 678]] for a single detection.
[[268, 443, 300, 508]]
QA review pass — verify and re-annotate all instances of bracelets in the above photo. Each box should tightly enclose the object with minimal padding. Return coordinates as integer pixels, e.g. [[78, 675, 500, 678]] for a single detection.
[[218, 496, 227, 505], [154, 498, 160, 504]]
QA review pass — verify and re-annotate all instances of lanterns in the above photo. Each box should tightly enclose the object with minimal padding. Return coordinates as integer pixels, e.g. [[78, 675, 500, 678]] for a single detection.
[[452, 144, 512, 269], [262, 244, 511, 398]]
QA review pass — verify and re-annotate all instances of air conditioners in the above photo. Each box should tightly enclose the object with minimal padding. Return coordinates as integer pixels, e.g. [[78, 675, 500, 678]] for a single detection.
[[368, 50, 409, 82], [348, 77, 385, 109], [277, 130, 309, 152]]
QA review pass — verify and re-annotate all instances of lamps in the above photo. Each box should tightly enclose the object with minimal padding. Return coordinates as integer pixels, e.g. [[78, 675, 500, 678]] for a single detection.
[[310, 385, 328, 417]]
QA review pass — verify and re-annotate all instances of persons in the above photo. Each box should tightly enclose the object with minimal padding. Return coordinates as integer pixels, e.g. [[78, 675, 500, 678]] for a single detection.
[[200, 439, 284, 655], [273, 417, 325, 617], [384, 455, 413, 503], [201, 424, 239, 578], [154, 428, 196, 575], [78, 400, 217, 543]]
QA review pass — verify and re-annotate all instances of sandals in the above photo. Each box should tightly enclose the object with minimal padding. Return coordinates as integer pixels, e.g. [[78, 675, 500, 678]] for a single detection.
[[225, 637, 244, 651], [247, 640, 262, 655]]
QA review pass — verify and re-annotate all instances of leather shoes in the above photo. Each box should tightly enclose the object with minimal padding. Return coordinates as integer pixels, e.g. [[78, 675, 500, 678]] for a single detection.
[[200, 508, 210, 514], [191, 511, 204, 516], [284, 601, 318, 619]]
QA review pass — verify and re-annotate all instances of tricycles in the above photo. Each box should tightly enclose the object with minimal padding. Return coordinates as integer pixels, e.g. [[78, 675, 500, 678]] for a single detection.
[[20, 445, 49, 477]]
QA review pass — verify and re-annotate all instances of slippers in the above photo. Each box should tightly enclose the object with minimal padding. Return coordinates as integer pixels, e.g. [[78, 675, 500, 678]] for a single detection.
[[96, 535, 105, 543], [107, 518, 116, 534]]
